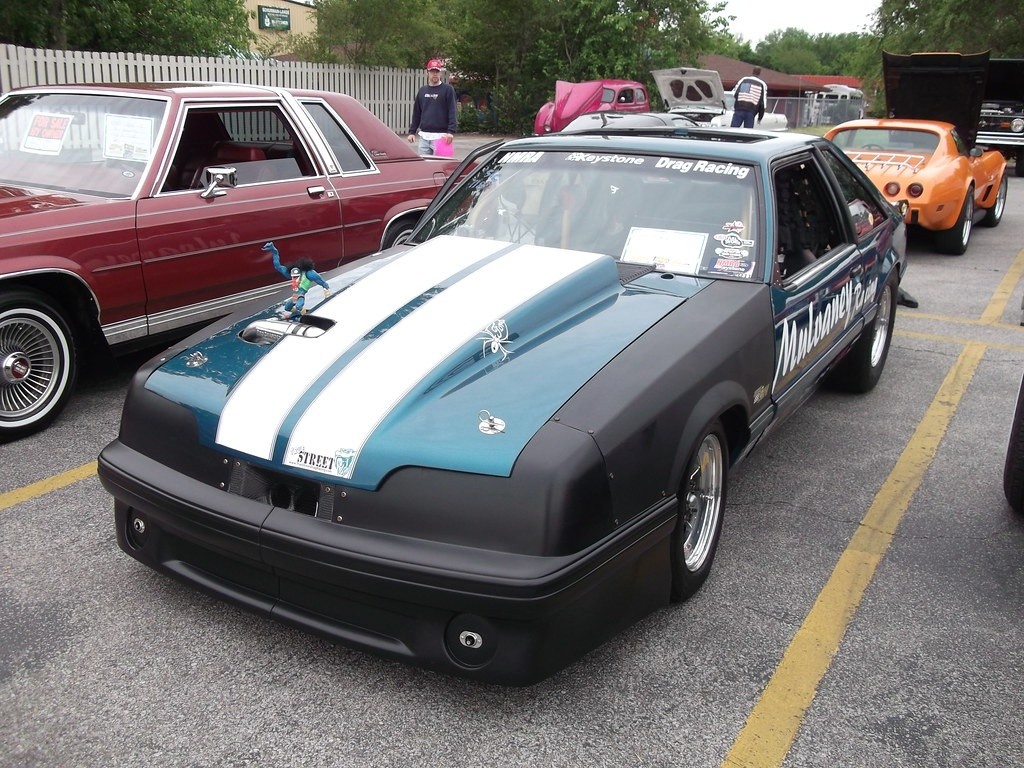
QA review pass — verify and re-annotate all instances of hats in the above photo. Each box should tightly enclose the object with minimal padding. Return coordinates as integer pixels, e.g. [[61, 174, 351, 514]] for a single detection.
[[427, 58, 441, 72]]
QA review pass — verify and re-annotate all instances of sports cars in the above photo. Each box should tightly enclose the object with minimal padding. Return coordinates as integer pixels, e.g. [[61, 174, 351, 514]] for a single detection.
[[822, 49, 1008, 256], [96, 127, 910, 686]]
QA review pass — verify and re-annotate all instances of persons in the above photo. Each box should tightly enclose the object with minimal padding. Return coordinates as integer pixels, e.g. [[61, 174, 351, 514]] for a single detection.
[[406, 60, 457, 161], [728, 67, 769, 134], [261, 242, 329, 323]]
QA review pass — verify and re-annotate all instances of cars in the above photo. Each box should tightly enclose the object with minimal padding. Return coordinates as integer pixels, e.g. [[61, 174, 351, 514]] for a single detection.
[[649, 68, 788, 130], [975, 59, 1024, 177], [534, 79, 650, 137], [2, 81, 477, 443], [542, 109, 701, 138]]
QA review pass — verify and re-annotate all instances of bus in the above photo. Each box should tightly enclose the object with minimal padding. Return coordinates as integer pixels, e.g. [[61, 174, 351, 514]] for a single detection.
[[812, 83, 865, 125]]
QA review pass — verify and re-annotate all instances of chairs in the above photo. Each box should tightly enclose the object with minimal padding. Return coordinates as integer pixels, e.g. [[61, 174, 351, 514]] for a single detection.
[[188, 142, 267, 191]]
